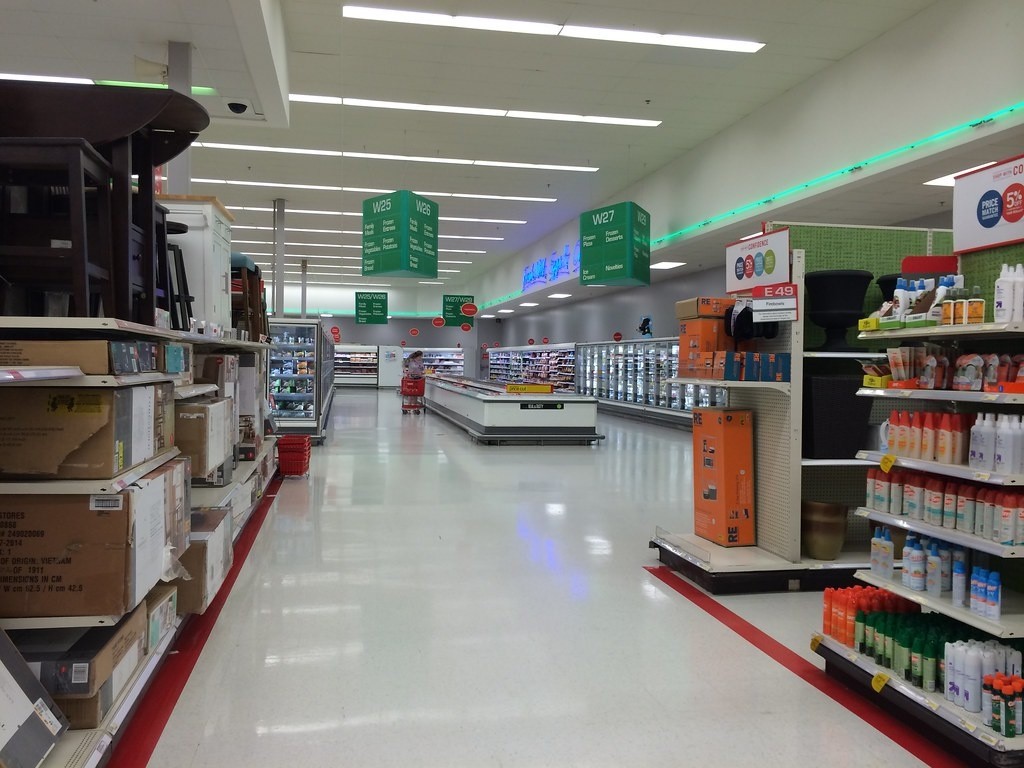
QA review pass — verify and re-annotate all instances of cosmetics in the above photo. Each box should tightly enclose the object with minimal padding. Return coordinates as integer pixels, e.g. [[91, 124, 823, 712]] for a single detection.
[[866, 467, 1024, 546], [892, 262, 1024, 328], [887, 410, 1024, 474]]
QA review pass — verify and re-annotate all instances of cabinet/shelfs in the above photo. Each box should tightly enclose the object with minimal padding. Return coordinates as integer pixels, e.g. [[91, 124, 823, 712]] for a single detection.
[[486, 344, 574, 393], [810, 321, 1024, 768], [156, 193, 235, 337], [334, 346, 378, 385], [267, 326, 317, 419], [0, 315, 279, 768], [652, 247, 884, 597], [404, 347, 464, 377]]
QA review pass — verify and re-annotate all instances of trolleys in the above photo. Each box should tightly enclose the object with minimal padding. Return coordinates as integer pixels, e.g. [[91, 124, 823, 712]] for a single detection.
[[401, 371, 427, 415]]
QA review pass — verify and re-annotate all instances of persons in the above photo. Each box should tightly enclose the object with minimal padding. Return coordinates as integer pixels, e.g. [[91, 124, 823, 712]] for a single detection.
[[404, 350, 424, 415]]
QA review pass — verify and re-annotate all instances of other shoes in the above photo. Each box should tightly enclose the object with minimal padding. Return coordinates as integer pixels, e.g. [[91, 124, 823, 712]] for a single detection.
[[403, 409, 408, 414], [415, 409, 421, 415]]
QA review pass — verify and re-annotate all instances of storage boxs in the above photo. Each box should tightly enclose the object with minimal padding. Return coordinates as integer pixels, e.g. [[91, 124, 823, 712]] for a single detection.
[[692, 407, 756, 548], [674, 298, 790, 381], [0, 340, 279, 729]]
